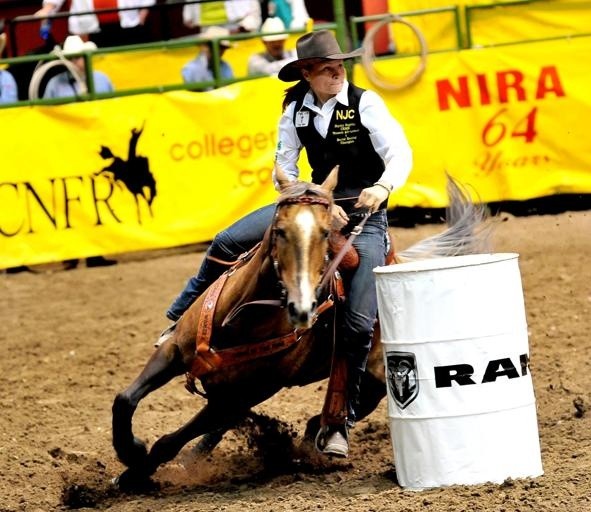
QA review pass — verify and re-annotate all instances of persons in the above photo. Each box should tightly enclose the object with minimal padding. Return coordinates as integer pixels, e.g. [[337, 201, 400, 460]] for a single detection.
[[155, 29, 415, 458]]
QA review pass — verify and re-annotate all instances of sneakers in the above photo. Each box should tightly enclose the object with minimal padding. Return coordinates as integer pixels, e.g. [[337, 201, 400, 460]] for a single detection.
[[323, 427, 349, 458], [8, 254, 118, 274], [158, 322, 183, 347]]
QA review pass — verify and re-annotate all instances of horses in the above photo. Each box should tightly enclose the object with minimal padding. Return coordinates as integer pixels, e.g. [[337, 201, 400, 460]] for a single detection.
[[109, 160, 494, 493]]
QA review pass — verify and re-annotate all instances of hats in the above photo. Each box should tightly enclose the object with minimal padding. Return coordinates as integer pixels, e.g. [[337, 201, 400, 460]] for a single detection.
[[261, 15, 288, 41], [59, 36, 97, 54], [278, 28, 367, 82], [196, 25, 232, 49]]
